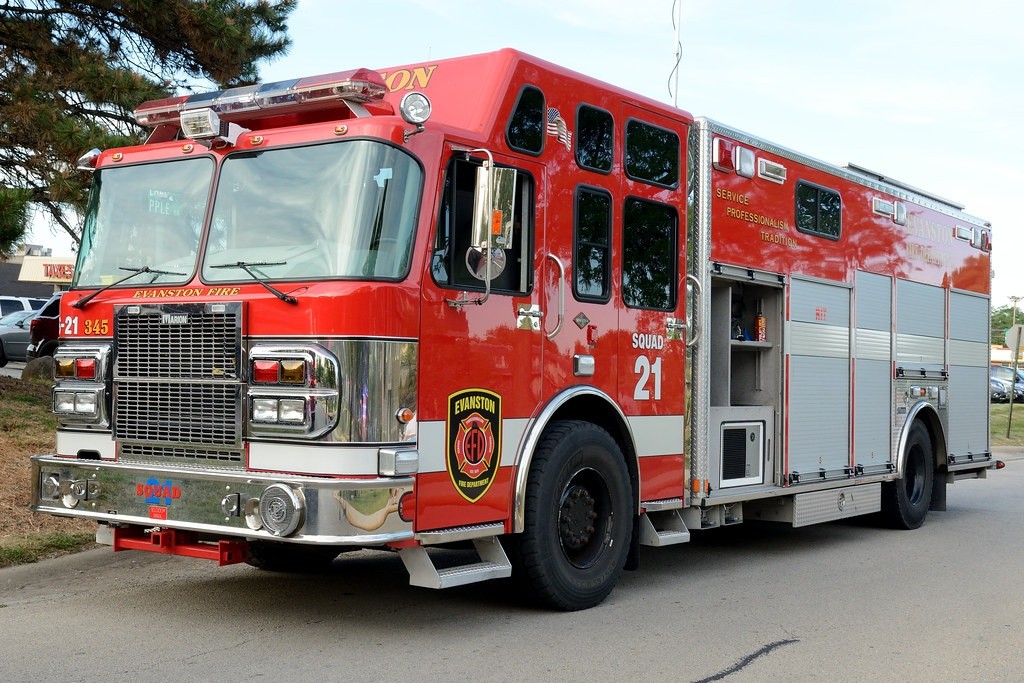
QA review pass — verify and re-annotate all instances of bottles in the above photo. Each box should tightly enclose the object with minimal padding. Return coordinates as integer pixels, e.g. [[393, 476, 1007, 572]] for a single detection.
[[754, 312, 768, 342]]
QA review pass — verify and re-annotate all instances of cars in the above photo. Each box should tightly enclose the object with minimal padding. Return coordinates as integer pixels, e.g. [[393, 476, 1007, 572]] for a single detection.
[[991, 365, 1024, 403], [0, 310, 41, 368]]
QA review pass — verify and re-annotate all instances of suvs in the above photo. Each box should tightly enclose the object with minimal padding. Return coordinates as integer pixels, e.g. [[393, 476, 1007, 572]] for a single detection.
[[26, 290, 67, 364], [0, 295, 49, 320]]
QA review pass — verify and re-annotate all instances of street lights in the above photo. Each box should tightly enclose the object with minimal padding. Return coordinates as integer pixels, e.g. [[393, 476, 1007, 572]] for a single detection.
[[1007, 295, 1024, 366]]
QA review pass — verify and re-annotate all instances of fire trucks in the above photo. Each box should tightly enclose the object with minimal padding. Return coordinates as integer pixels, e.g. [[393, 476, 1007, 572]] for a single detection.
[[29, 48, 1007, 615]]
